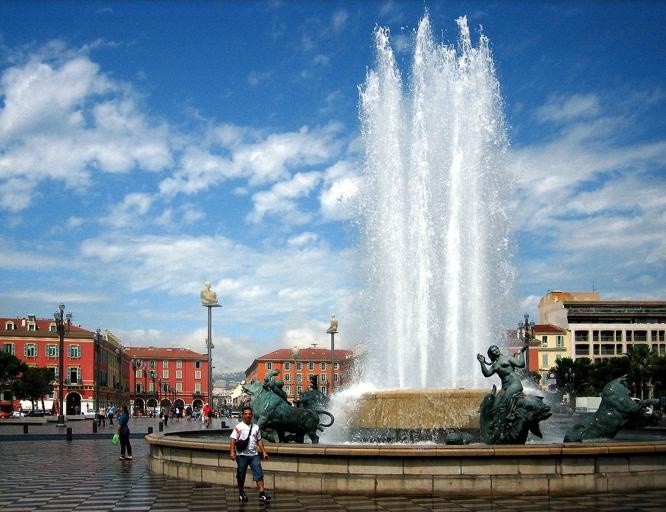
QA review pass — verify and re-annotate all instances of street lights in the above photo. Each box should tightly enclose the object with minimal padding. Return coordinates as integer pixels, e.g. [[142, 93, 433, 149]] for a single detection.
[[116, 347, 176, 417], [93, 329, 102, 418], [519, 313, 535, 372], [53, 303, 71, 424]]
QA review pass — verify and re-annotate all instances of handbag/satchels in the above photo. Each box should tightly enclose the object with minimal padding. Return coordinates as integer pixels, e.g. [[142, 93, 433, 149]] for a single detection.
[[112, 432, 120, 445], [236, 438, 249, 452]]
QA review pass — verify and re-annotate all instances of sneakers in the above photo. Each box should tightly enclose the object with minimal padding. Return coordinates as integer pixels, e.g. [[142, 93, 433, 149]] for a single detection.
[[259, 493, 271, 502], [120, 455, 133, 460], [240, 490, 248, 501]]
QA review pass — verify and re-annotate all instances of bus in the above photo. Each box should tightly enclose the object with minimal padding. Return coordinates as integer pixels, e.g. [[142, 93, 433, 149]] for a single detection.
[[0, 399, 13, 419]]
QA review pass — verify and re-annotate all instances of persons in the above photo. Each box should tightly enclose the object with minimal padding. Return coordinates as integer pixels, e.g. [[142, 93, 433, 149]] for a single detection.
[[115, 403, 133, 460], [97, 401, 232, 428], [201, 281, 217, 305], [262, 369, 287, 402], [477, 345, 527, 428], [229, 406, 272, 501], [326, 315, 337, 331]]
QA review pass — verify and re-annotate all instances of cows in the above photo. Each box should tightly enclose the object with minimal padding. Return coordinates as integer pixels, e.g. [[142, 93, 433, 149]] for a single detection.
[[242, 380, 334, 445]]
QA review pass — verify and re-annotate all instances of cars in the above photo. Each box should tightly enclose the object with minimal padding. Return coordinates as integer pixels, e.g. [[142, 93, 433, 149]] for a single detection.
[[11, 410, 51, 419]]
